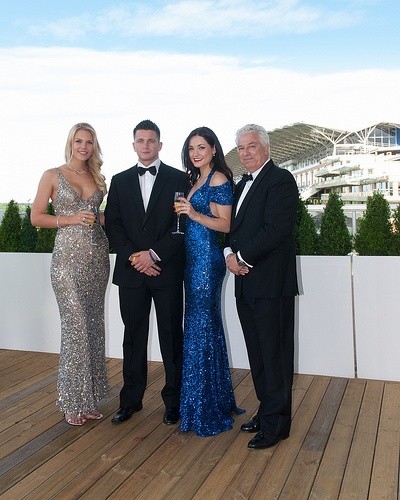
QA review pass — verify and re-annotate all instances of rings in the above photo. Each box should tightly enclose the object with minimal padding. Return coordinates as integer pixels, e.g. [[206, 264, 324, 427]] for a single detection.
[[82, 217, 86, 222]]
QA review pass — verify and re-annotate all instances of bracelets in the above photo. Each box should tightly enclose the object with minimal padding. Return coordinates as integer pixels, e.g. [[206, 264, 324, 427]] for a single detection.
[[149, 250, 157, 263], [56, 214, 62, 229]]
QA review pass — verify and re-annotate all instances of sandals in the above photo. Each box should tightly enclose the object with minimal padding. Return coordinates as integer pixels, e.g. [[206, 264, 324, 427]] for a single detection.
[[63, 410, 103, 425]]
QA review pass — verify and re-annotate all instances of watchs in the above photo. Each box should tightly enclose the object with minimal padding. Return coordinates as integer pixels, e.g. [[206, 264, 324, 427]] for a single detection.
[[235, 254, 245, 267]]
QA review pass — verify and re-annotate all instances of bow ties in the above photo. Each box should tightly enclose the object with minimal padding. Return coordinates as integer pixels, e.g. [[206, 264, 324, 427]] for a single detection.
[[242, 174, 253, 182], [137, 166, 156, 176]]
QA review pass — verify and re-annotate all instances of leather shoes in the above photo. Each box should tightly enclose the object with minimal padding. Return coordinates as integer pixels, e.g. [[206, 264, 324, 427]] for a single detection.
[[248, 429, 289, 449], [163, 406, 180, 424], [111, 405, 143, 422], [241, 415, 260, 433]]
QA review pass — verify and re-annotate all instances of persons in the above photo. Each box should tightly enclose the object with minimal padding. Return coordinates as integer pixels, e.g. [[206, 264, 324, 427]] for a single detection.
[[29, 122, 110, 426], [223, 125, 299, 449], [104, 119, 194, 425], [180, 127, 247, 438]]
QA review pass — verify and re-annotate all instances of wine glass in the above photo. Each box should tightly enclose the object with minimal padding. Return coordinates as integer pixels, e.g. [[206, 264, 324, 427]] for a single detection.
[[86, 205, 99, 246], [172, 192, 184, 235]]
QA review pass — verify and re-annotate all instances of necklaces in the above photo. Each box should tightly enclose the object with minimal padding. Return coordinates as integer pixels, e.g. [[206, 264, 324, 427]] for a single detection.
[[65, 164, 89, 174]]
